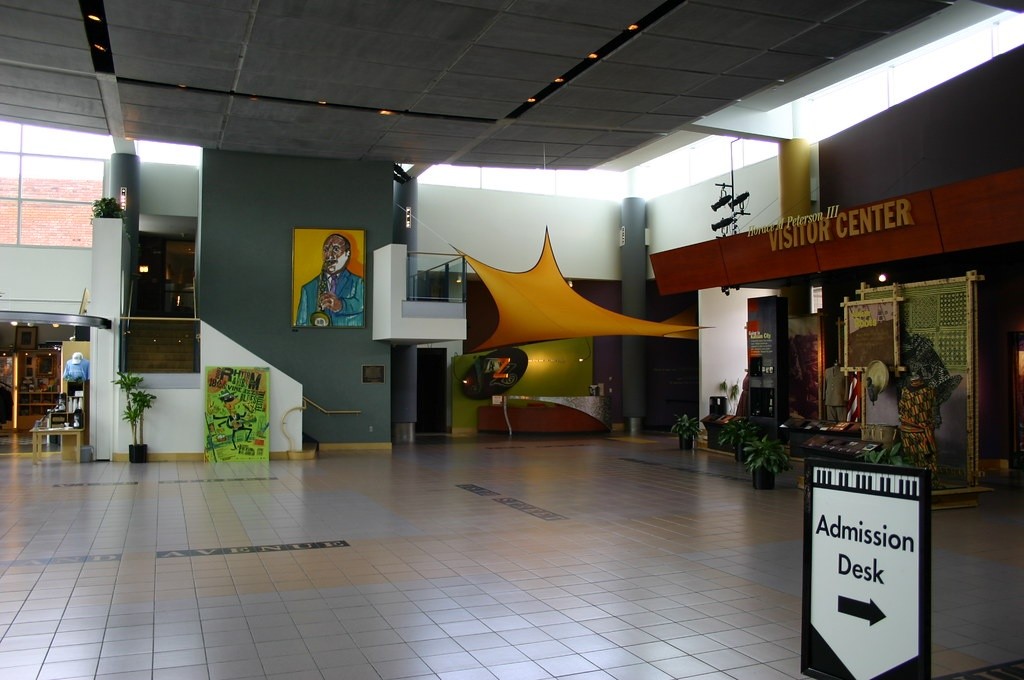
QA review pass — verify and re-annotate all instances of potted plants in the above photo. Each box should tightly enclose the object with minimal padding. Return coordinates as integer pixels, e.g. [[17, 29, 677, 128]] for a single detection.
[[670, 413, 702, 450], [742, 434, 793, 490], [95, 198, 122, 218], [114, 372, 156, 463]]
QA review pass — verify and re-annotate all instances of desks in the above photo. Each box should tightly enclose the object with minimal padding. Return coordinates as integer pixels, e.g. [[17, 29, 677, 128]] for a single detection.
[[29, 426, 84, 464], [478, 394, 612, 432]]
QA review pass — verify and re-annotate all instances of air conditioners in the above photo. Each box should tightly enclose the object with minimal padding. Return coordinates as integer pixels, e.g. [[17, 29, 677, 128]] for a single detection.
[[716, 418, 757, 462]]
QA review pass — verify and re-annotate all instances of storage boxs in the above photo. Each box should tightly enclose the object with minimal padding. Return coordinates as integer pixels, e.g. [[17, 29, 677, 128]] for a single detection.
[[598, 383, 605, 397], [986, 459, 1009, 471]]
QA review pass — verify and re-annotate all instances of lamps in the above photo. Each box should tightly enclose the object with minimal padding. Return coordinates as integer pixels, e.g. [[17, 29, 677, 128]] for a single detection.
[[711, 195, 732, 212], [728, 191, 750, 209], [459, 375, 470, 385], [711, 217, 734, 232], [394, 163, 415, 188]]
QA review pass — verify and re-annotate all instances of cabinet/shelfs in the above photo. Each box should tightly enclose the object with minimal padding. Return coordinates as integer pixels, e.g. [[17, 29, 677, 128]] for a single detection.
[[60, 340, 90, 396], [18, 392, 60, 430]]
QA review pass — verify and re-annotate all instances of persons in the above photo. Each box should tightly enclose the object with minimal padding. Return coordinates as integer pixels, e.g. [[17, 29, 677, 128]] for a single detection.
[[822, 365, 851, 422], [62, 352, 90, 429], [898, 375, 941, 486]]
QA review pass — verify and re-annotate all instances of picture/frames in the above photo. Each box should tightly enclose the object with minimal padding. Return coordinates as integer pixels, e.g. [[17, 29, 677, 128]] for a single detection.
[[291, 227, 367, 330], [15, 325, 38, 350], [361, 365, 386, 384], [840, 296, 906, 378], [35, 353, 57, 378]]
